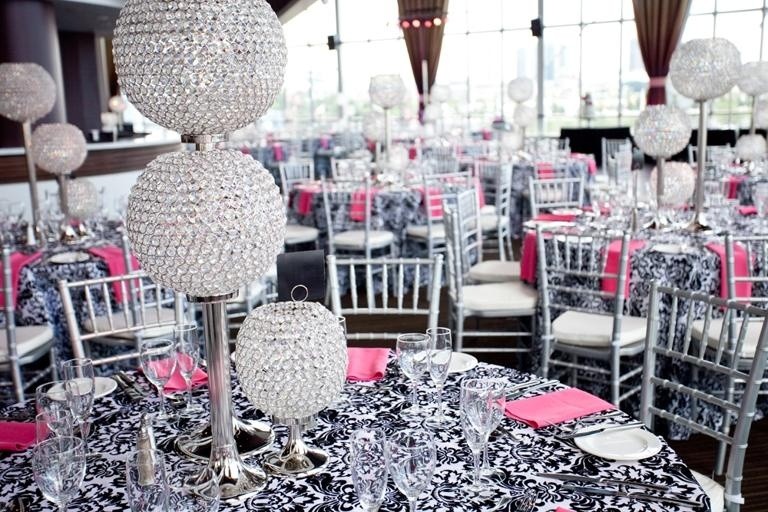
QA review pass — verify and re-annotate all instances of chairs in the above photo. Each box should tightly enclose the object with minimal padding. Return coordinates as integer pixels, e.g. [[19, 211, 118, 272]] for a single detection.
[[1, 113, 768, 512]]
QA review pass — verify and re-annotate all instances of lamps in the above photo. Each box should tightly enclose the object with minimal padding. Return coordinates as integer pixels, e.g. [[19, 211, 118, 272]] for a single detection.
[[369, 73, 533, 180], [1, 62, 103, 251], [112, 1, 290, 496], [632, 39, 768, 213]]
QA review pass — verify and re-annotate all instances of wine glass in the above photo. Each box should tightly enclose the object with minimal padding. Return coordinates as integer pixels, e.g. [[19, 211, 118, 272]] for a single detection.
[[348, 324, 505, 512], [523, 146, 768, 241], [32, 326, 221, 512]]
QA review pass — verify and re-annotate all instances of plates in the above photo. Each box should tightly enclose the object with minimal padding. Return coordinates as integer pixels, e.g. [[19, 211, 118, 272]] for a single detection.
[[576, 426, 661, 461]]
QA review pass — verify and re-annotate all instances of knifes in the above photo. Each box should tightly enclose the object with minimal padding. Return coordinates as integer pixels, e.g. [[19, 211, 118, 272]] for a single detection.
[[562, 422, 645, 440], [508, 380, 560, 400], [535, 472, 670, 492], [559, 483, 705, 508]]
